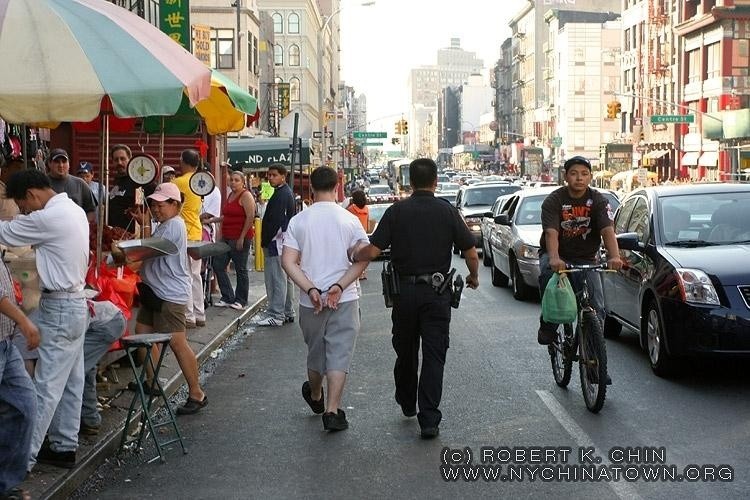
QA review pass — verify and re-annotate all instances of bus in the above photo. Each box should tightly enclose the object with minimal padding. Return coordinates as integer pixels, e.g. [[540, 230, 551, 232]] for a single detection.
[[388, 159, 404, 190], [392, 162, 413, 198]]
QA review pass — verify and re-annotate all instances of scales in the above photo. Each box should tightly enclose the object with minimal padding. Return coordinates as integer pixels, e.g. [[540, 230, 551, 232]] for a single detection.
[[115, 152, 179, 262], [187, 157, 233, 260]]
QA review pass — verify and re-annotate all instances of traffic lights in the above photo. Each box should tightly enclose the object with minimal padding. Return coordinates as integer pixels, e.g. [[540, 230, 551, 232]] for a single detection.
[[607, 100, 621, 119], [395, 120, 401, 134], [402, 120, 408, 135]]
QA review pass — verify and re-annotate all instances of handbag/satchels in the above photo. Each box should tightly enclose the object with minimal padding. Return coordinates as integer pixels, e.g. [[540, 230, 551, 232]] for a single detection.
[[543, 267, 577, 324]]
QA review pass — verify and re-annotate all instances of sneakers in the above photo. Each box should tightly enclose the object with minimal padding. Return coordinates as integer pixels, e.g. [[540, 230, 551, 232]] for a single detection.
[[303, 381, 325, 414], [322, 408, 349, 431], [79, 424, 101, 436], [185, 321, 205, 329], [592, 369, 613, 384], [536, 315, 560, 347], [231, 302, 248, 310], [215, 301, 230, 308], [395, 391, 417, 415], [422, 425, 439, 439], [256, 316, 283, 326], [38, 447, 77, 468], [284, 316, 295, 322]]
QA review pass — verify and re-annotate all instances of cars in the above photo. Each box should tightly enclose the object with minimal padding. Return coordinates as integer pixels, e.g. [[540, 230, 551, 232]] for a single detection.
[[368, 184, 394, 196], [490, 186, 622, 301], [481, 193, 514, 265], [367, 172, 379, 183], [342, 193, 403, 257], [595, 183, 750, 376]]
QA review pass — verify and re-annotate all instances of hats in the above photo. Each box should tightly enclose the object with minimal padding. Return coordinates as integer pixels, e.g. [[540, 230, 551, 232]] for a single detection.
[[563, 157, 593, 173], [76, 162, 94, 174], [51, 148, 70, 160], [148, 183, 182, 203]]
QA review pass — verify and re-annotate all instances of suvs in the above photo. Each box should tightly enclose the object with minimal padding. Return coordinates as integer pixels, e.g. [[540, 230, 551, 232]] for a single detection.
[[453, 181, 522, 258]]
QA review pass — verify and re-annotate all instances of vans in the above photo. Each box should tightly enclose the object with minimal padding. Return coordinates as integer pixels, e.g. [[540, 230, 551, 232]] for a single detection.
[[438, 183, 460, 201], [437, 175, 449, 183]]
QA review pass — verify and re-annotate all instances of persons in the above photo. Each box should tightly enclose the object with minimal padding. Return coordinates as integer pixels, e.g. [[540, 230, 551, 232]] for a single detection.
[[0, 154, 26, 223], [256, 163, 298, 327], [348, 158, 479, 441], [12, 301, 128, 434], [537, 156, 623, 385], [170, 148, 206, 329], [197, 162, 222, 310], [159, 165, 176, 183], [74, 160, 109, 229], [46, 148, 99, 223], [111, 183, 209, 415], [0, 244, 41, 500], [280, 166, 371, 431], [105, 144, 138, 233], [345, 191, 370, 282], [213, 171, 255, 312], [0, 168, 91, 478]]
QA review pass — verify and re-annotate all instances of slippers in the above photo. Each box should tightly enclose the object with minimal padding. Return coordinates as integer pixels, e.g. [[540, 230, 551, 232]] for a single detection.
[[128, 381, 165, 396], [176, 395, 208, 413]]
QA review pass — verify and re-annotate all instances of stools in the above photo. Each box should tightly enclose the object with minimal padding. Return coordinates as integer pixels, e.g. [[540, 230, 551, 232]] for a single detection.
[[118, 335, 189, 463]]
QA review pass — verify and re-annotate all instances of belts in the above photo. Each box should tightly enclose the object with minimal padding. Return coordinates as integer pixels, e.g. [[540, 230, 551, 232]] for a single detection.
[[40, 291, 87, 298], [401, 274, 433, 284]]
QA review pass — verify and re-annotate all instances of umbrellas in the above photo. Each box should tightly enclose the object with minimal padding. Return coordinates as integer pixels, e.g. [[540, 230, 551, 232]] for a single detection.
[[0, 0, 213, 268], [74, 63, 260, 179]]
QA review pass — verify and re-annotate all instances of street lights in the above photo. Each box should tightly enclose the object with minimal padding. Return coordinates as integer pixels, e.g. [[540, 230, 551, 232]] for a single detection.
[[321, 1, 376, 160]]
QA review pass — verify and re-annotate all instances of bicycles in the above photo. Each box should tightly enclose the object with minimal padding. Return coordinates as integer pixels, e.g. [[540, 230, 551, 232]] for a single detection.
[[537, 261, 630, 412]]
